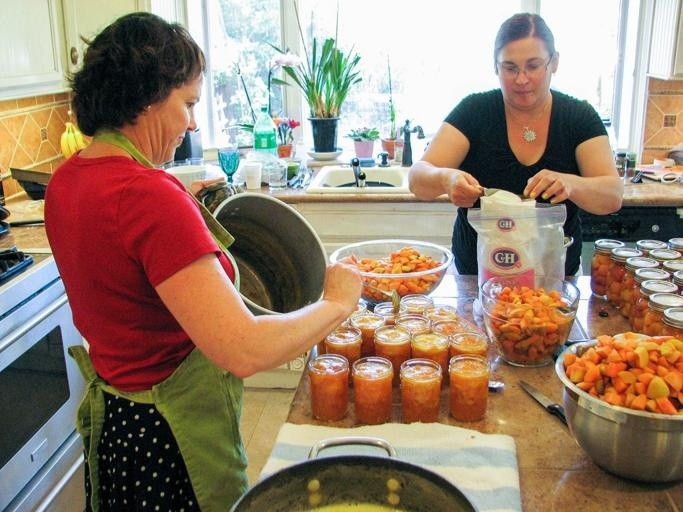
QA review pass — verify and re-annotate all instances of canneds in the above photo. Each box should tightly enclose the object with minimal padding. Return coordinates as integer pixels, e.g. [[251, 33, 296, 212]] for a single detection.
[[591, 235, 682, 339], [306, 293, 490, 424]]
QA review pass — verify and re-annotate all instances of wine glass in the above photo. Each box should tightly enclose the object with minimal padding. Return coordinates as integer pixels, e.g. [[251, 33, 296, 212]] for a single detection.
[[217, 149, 239, 186]]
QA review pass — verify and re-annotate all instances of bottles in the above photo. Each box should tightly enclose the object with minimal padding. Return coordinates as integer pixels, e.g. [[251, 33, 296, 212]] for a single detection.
[[253, 106, 275, 160], [615, 151, 636, 178], [589, 237, 682, 340]]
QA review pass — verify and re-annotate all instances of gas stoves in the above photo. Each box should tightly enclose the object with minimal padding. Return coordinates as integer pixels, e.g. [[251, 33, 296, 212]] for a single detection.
[[0, 248, 54, 290]]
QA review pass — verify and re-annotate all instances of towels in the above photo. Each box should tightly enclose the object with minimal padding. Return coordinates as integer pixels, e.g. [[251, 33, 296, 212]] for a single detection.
[[257, 420, 523, 512]]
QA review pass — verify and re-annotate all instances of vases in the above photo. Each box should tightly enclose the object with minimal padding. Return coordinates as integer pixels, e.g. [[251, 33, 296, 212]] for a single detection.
[[276, 143, 293, 160]]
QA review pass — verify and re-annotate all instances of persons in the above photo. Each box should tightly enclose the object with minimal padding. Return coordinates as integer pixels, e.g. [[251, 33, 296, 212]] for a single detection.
[[43, 10, 365, 511], [407, 12, 625, 276]]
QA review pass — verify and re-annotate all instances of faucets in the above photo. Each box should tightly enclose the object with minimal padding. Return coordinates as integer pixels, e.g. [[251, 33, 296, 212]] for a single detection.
[[401, 119, 425, 166]]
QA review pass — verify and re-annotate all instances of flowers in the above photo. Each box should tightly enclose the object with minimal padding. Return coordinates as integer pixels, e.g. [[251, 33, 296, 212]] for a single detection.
[[231, 53, 302, 146]]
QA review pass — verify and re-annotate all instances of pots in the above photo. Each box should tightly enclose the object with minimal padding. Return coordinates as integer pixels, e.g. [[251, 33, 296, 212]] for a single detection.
[[228, 436, 479, 512], [194, 182, 327, 317]]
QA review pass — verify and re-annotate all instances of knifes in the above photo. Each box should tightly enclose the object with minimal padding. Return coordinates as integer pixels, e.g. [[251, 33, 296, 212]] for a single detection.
[[515, 381, 571, 429]]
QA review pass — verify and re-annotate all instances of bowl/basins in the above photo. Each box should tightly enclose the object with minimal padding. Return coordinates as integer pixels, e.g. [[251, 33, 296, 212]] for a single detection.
[[330, 239, 454, 308], [164, 165, 206, 190], [480, 274, 579, 368], [553, 339, 682, 485]]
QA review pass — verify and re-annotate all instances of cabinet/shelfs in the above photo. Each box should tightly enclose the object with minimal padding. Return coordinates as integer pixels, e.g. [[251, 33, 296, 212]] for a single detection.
[[61, 0, 175, 92], [2, 2, 65, 102]]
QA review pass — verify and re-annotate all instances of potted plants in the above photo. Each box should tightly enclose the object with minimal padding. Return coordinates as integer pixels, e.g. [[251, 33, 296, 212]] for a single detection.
[[265, 33, 363, 152], [345, 125, 378, 158]]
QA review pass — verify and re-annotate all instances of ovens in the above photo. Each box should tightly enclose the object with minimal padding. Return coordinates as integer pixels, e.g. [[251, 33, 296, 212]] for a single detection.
[[0, 276, 85, 512]]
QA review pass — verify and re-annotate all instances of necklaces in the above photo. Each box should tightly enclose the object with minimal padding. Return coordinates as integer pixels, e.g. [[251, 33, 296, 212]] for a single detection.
[[504, 102, 552, 142]]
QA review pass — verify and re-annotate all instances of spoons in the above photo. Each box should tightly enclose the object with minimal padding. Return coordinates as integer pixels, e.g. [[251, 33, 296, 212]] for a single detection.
[[361, 281, 399, 314]]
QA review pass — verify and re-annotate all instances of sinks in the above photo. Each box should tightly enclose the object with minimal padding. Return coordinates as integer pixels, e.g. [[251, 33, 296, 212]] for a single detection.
[[306, 165, 410, 193]]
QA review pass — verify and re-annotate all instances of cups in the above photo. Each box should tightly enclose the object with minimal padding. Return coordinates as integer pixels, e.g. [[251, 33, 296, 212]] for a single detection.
[[307, 294, 492, 424], [267, 159, 287, 188], [242, 163, 262, 190]]
